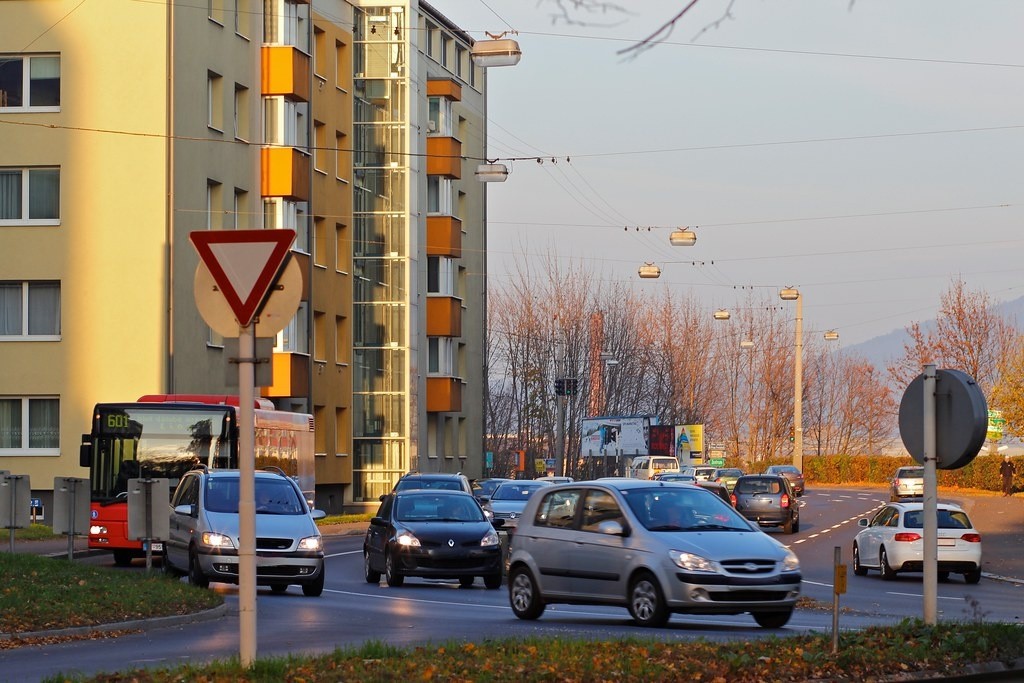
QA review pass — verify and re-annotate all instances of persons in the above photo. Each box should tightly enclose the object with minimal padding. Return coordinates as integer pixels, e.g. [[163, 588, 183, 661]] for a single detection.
[[999, 455, 1016, 497]]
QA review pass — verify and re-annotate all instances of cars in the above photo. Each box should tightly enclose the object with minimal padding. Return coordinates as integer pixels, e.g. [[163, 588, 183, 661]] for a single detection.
[[651, 473, 698, 483], [468, 477, 510, 504], [889, 466, 925, 503], [710, 468, 746, 495], [535, 476, 578, 507], [768, 466, 804, 497], [730, 473, 800, 533], [364, 489, 504, 589], [852, 502, 983, 583], [507, 477, 803, 628], [479, 479, 573, 532], [690, 481, 732, 507], [682, 466, 716, 481]]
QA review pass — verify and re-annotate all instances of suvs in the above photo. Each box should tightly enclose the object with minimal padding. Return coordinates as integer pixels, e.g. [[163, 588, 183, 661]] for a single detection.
[[163, 463, 326, 597], [379, 470, 473, 496]]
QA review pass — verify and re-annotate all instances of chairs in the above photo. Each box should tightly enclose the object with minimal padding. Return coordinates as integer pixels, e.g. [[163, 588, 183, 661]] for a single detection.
[[206, 488, 231, 512], [114, 460, 141, 493]]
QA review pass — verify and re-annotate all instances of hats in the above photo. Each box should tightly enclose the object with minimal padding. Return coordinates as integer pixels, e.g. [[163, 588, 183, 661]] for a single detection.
[[1005, 456, 1010, 458]]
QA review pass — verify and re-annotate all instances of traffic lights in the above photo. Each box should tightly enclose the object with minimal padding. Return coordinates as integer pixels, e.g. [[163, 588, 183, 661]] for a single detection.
[[559, 379, 565, 395], [790, 427, 795, 442], [573, 379, 578, 395], [566, 379, 572, 395], [554, 380, 561, 395]]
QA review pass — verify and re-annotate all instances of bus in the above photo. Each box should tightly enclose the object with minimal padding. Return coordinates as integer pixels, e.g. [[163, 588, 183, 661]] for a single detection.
[[628, 456, 680, 479], [79, 394, 316, 564]]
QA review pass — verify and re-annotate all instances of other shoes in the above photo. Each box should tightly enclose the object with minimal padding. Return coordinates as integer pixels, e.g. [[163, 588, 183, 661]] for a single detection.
[[1007, 494, 1010, 497], [1002, 493, 1006, 497]]
